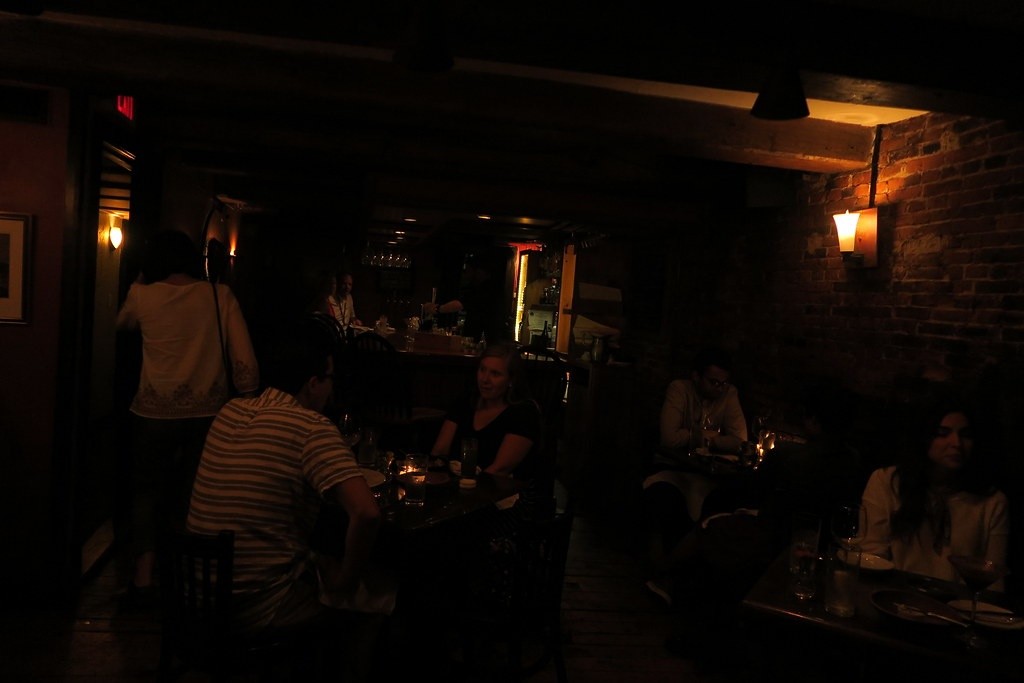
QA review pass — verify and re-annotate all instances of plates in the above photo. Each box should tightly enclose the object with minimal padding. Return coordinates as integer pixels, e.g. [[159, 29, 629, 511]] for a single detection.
[[839, 553, 894, 573], [947, 598, 1024, 630], [871, 589, 959, 627], [360, 468, 387, 487]]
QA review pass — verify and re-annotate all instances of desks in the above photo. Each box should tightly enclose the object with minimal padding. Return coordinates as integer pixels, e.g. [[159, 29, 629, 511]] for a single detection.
[[649, 442, 846, 597], [737, 545, 1024, 683], [354, 327, 484, 407], [356, 452, 532, 683]]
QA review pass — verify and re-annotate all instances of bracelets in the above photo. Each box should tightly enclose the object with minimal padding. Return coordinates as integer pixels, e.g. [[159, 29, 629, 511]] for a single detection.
[[345, 551, 371, 562]]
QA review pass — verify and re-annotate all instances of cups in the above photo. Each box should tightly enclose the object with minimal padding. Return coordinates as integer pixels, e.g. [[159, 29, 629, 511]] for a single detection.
[[461, 439, 478, 478], [827, 543, 863, 620], [340, 422, 361, 449], [404, 453, 428, 509], [359, 431, 378, 468], [787, 512, 822, 598]]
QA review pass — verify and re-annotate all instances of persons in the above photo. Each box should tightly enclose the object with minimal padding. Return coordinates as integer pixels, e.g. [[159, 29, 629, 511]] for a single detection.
[[208, 237, 240, 292], [850, 401, 1008, 683], [423, 278, 466, 336], [644, 344, 862, 652], [322, 270, 363, 338], [378, 342, 539, 660], [116, 228, 260, 602], [170, 314, 391, 683]]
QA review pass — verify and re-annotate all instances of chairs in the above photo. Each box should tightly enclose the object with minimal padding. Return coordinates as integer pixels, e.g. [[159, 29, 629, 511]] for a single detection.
[[157, 310, 862, 683]]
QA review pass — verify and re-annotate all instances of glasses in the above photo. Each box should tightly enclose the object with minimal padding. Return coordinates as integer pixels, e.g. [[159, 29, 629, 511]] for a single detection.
[[703, 376, 727, 387]]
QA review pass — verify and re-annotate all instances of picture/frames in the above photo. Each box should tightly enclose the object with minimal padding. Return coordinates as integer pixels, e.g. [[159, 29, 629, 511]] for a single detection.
[[0, 211, 33, 327]]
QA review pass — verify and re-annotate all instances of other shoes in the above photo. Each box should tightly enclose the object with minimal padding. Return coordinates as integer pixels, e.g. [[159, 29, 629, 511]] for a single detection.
[[646, 577, 672, 605]]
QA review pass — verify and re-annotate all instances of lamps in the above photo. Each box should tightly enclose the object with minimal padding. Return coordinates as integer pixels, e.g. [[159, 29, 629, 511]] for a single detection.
[[832, 208, 864, 276], [360, 244, 412, 273], [109, 227, 122, 250], [751, 68, 809, 121]]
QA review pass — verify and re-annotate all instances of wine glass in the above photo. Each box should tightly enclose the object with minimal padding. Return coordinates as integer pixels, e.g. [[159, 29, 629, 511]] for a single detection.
[[832, 504, 869, 580]]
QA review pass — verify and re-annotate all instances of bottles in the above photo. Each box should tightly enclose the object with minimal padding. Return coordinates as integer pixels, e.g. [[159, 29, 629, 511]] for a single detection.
[[540, 321, 549, 353]]
